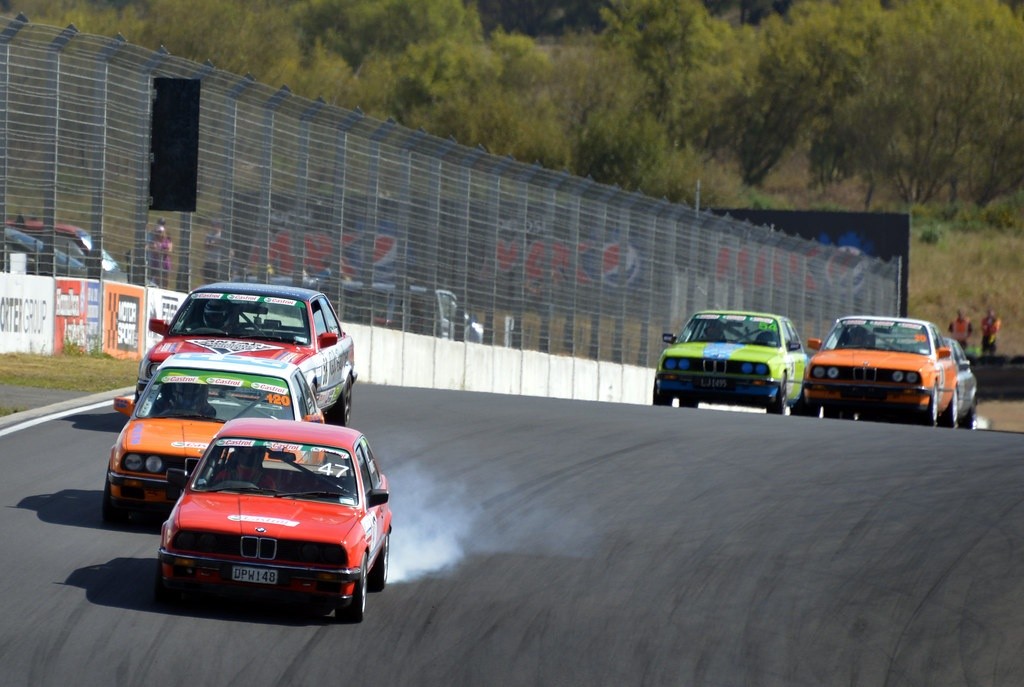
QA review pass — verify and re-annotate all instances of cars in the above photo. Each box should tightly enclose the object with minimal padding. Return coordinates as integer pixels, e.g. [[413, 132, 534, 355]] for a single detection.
[[154, 419, 394, 622], [0, 217, 486, 343], [98, 352, 330, 524], [653, 308, 811, 415], [134, 283, 358, 425], [801, 312, 981, 429]]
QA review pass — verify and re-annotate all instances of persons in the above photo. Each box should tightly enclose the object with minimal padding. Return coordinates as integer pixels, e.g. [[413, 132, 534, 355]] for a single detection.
[[845, 325, 872, 349], [148, 376, 217, 420], [183, 297, 244, 341], [980, 307, 1002, 356], [213, 445, 278, 498], [134, 208, 265, 287], [946, 306, 972, 355], [703, 319, 724, 341]]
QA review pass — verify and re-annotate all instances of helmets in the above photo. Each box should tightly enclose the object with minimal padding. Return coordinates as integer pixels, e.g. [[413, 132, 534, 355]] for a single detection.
[[170, 382, 203, 410], [702, 322, 723, 338], [229, 448, 263, 484], [203, 299, 230, 328], [846, 326, 867, 344]]
